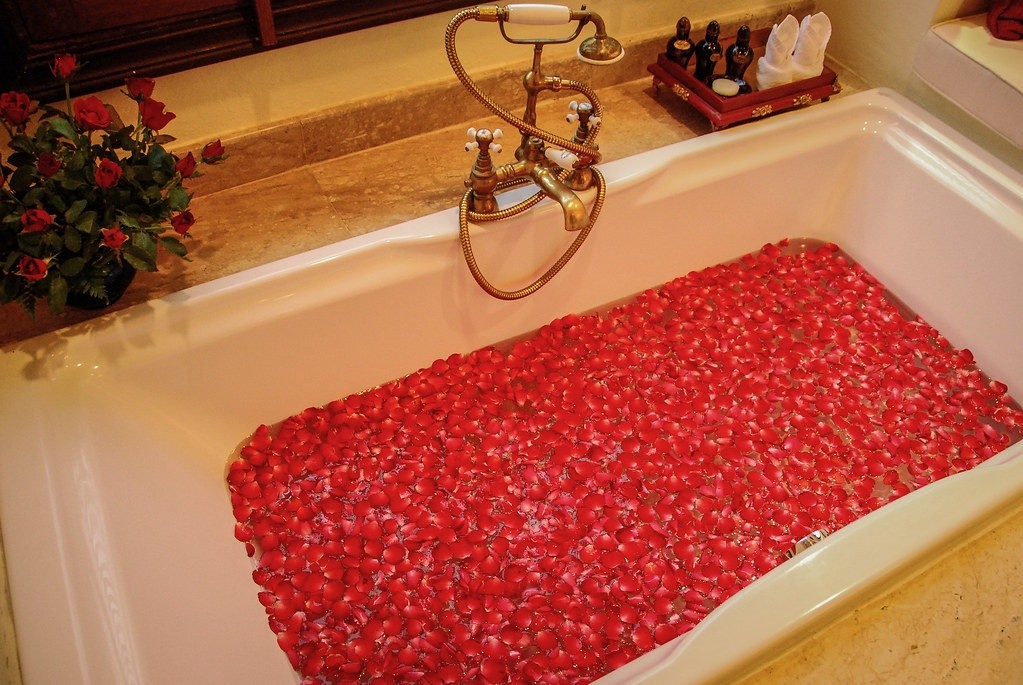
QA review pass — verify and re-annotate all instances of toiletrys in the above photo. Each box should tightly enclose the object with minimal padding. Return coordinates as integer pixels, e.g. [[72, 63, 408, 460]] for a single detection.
[[665, 15, 755, 84]]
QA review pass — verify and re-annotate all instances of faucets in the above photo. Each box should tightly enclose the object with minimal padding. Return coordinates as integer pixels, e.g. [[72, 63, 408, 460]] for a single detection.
[[515, 134, 592, 232]]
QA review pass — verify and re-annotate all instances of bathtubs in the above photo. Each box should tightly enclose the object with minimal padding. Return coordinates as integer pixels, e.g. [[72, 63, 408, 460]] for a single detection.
[[1, 83, 1022, 685]]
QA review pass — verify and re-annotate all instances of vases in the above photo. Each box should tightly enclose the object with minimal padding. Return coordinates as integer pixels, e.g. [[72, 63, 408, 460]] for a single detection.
[[60, 260, 137, 310]]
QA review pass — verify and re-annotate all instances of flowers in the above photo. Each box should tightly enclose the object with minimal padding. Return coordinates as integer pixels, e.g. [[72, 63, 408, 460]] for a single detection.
[[0, 50, 230, 328]]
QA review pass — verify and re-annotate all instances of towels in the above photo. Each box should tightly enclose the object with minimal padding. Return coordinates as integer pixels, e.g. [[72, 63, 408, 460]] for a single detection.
[[756, 13, 799, 91], [792, 11, 832, 82]]
